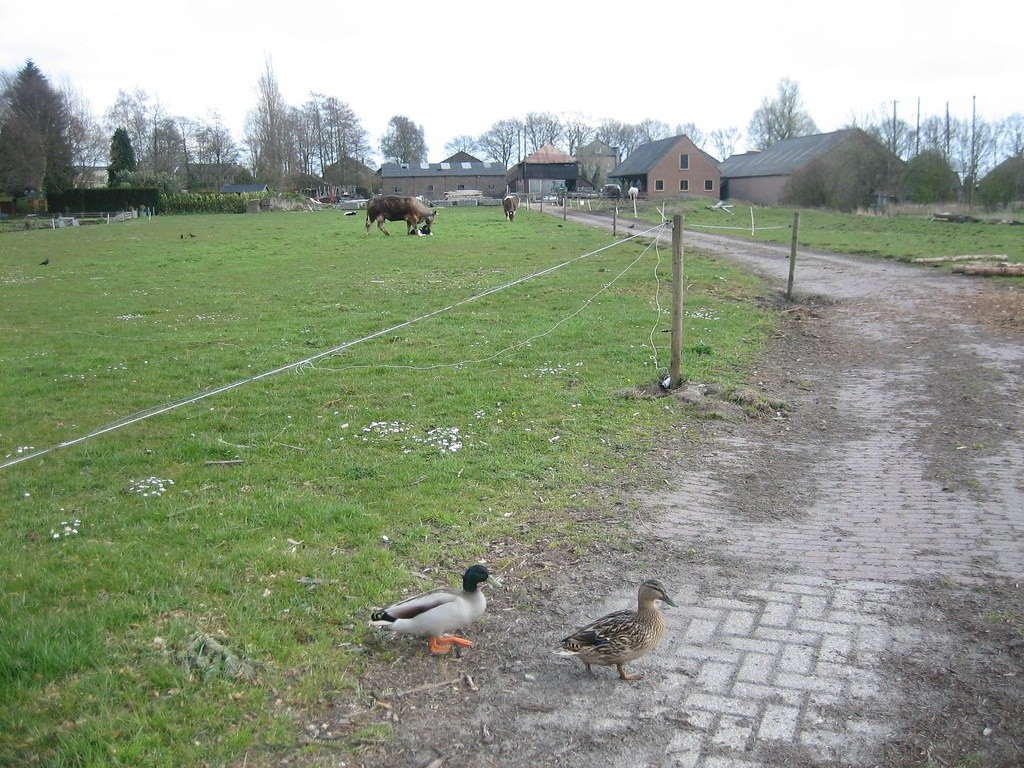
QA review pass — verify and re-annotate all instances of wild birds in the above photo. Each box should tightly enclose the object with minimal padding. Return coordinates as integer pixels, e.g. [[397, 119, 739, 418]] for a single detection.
[[180, 233, 184, 239], [39, 257, 49, 266], [628, 224, 635, 228], [189, 232, 197, 237]]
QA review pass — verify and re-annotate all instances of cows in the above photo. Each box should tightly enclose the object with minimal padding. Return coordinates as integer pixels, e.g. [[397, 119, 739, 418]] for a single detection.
[[502, 192, 520, 222], [365, 195, 441, 236], [599, 184, 641, 201]]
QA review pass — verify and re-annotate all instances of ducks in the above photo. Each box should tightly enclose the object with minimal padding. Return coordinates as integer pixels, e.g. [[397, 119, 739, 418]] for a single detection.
[[367, 564, 503, 656], [559, 579, 679, 680]]
